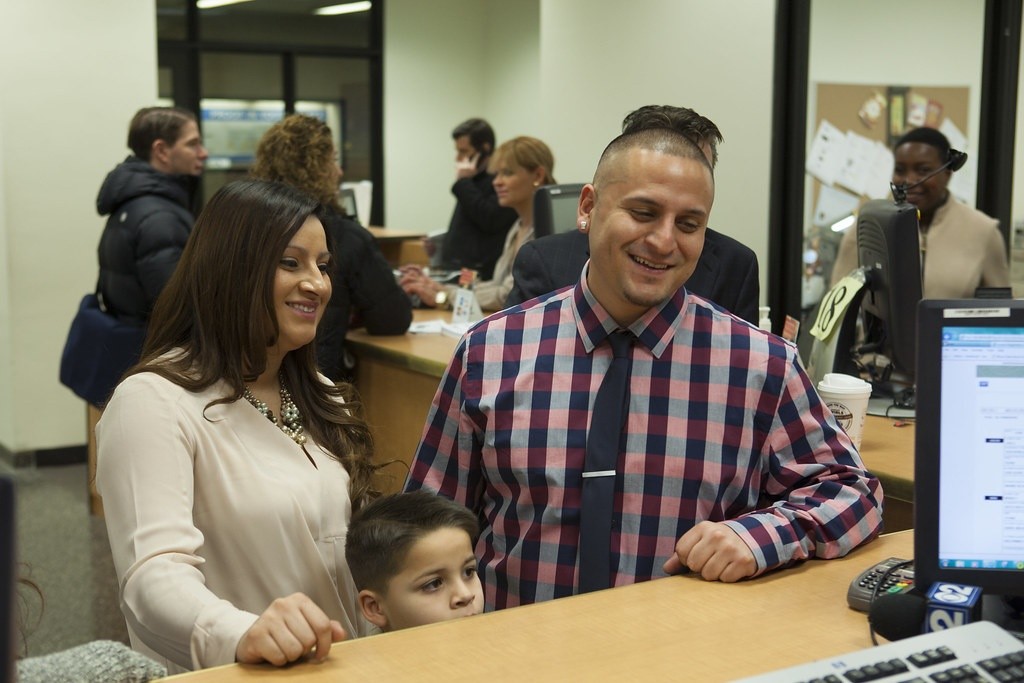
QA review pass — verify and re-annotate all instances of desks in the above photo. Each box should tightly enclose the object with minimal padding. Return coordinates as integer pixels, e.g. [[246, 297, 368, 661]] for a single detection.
[[343, 303, 921, 532], [138, 519, 917, 683]]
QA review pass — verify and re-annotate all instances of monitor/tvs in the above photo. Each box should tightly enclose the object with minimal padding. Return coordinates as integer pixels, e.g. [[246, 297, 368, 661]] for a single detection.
[[534, 183, 590, 239], [912, 299, 1024, 636], [804, 196, 923, 392]]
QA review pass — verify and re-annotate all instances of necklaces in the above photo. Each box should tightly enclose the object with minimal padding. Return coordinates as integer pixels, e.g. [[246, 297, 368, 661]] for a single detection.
[[248, 371, 306, 445]]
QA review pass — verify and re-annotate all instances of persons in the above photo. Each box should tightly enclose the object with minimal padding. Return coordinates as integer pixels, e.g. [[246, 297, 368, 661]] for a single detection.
[[95, 180, 380, 669], [97, 108, 761, 378], [405, 129, 884, 616], [343, 492, 486, 631], [825, 125, 1015, 384]]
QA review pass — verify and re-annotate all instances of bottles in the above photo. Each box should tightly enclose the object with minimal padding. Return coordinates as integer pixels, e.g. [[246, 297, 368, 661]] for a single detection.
[[759, 305, 772, 331]]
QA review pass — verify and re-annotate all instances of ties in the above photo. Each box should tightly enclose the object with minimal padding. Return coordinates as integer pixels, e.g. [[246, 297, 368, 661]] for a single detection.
[[577, 332, 634, 593]]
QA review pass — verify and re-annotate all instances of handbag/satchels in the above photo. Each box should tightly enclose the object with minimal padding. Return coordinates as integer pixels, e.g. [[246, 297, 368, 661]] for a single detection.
[[58, 291, 149, 411]]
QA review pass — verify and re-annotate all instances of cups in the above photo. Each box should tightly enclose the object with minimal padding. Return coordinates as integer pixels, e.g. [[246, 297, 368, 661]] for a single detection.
[[817, 373, 873, 452]]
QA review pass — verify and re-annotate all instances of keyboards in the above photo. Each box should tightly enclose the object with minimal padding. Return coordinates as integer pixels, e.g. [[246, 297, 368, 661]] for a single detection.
[[733, 620, 1024, 683]]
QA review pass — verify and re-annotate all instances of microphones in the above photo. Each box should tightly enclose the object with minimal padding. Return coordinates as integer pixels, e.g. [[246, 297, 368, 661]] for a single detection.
[[869, 581, 983, 644]]
